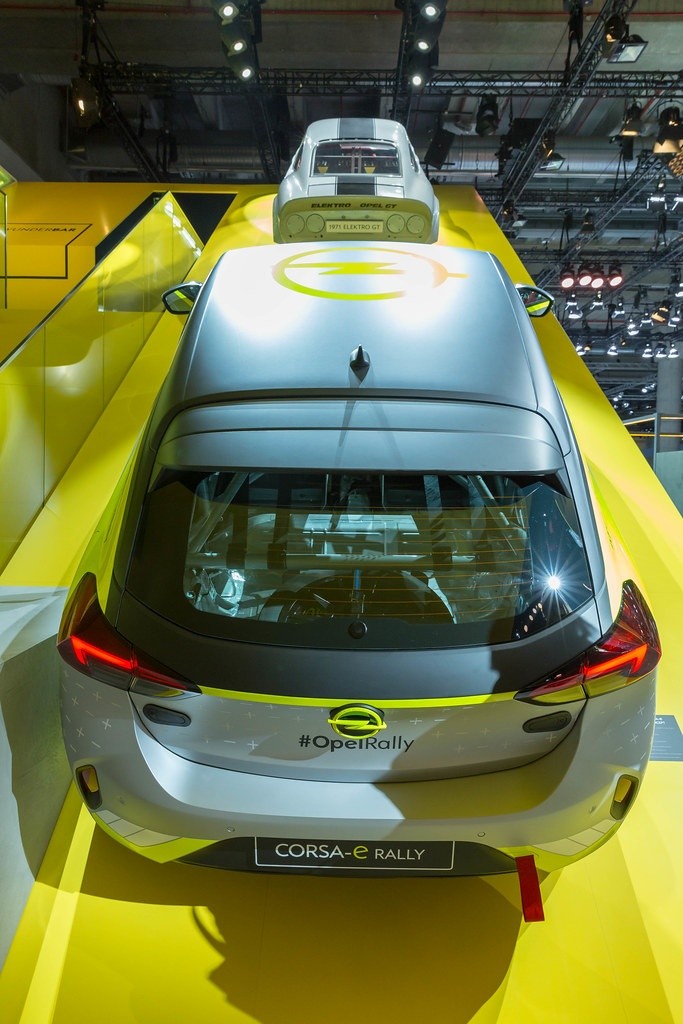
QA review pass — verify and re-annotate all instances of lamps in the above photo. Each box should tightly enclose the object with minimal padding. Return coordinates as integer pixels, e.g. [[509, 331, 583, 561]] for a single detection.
[[544, 153, 566, 172], [564, 294, 683, 359], [604, 18, 648, 63], [408, 0, 448, 88], [211, 0, 255, 84], [559, 263, 625, 289], [621, 103, 642, 135], [475, 94, 500, 136], [646, 192, 667, 212], [652, 106, 683, 153], [509, 214, 528, 228]]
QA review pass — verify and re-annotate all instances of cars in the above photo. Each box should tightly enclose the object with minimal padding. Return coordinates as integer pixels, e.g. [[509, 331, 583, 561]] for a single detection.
[[57, 240, 662, 881], [272, 117, 439, 245]]
